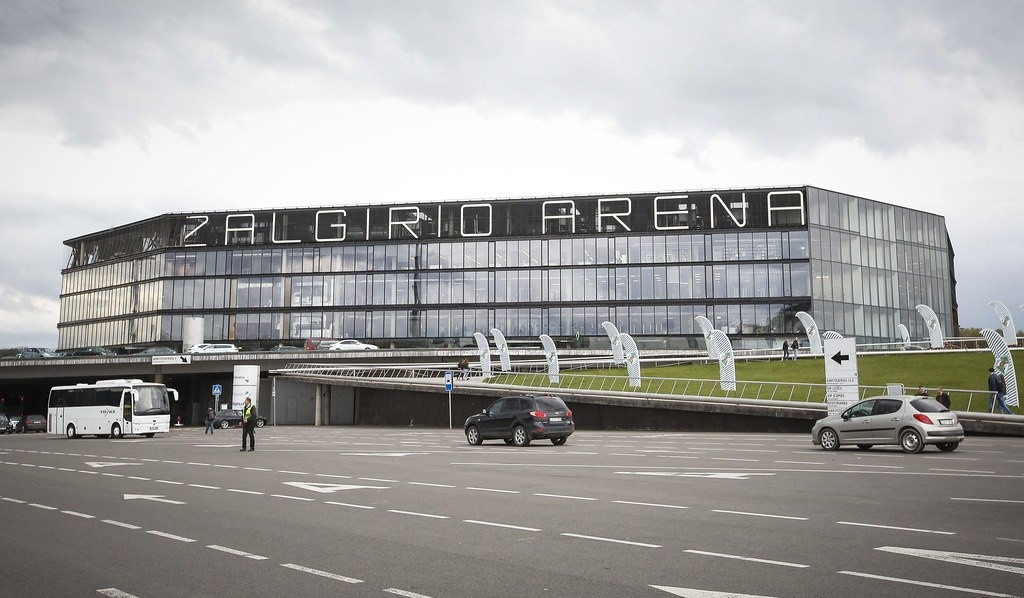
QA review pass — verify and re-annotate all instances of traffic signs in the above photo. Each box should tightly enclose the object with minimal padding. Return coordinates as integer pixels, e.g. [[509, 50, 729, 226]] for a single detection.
[[152, 355, 192, 365]]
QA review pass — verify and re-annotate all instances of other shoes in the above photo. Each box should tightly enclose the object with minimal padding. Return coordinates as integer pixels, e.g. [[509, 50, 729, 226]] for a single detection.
[[247, 448, 254, 452], [240, 449, 247, 451]]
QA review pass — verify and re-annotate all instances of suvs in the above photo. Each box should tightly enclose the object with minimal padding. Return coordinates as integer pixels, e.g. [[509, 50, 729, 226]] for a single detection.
[[464, 393, 575, 447], [188, 343, 239, 353], [304, 337, 339, 350]]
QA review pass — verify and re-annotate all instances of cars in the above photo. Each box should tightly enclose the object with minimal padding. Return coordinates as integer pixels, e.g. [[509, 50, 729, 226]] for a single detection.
[[133, 347, 177, 355], [16, 348, 116, 358], [812, 395, 965, 451], [0, 414, 47, 435], [213, 409, 268, 429], [270, 346, 304, 351], [328, 339, 380, 350]]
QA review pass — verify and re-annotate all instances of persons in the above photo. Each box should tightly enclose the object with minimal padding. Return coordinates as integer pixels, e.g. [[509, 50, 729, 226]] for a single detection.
[[988, 368, 1013, 414], [238, 397, 257, 452], [457, 359, 469, 381], [791, 337, 799, 360], [935, 386, 951, 409], [915, 386, 929, 396], [203, 406, 214, 435], [783, 340, 789, 360]]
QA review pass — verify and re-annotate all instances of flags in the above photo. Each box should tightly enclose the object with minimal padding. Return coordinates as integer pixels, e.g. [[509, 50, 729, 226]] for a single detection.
[[600, 322, 623, 364], [979, 329, 1020, 407], [915, 305, 944, 348], [490, 329, 511, 371], [795, 311, 843, 354], [694, 316, 736, 391], [618, 333, 641, 387], [473, 332, 491, 377], [989, 301, 1018, 346], [540, 334, 558, 383], [897, 324, 911, 349]]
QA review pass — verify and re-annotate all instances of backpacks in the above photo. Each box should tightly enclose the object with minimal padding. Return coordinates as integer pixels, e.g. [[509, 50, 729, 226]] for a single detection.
[[208, 410, 215, 420]]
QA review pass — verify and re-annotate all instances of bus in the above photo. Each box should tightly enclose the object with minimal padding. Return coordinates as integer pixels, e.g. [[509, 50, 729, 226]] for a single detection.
[[46, 379, 178, 439]]
